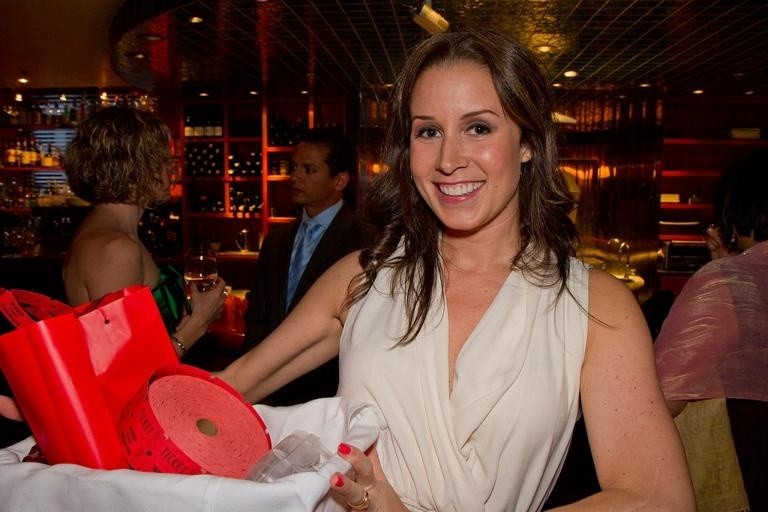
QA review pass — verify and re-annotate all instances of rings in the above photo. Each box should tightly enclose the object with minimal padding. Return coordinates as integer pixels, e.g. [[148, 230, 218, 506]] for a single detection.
[[348, 490, 371, 510]]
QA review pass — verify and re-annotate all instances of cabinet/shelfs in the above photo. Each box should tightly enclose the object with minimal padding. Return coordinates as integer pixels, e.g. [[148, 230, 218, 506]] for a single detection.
[[658, 94, 768, 290], [1, 85, 316, 274]]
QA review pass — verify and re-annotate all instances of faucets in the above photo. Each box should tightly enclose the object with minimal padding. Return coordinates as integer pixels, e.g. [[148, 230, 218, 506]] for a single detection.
[[604, 236, 619, 251], [617, 241, 630, 276]]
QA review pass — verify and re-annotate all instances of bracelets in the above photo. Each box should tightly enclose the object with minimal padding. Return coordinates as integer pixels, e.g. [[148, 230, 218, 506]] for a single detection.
[[171, 336, 188, 355]]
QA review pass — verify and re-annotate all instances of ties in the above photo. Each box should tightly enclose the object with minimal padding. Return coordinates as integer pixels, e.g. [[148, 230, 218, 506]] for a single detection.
[[287, 221, 325, 311]]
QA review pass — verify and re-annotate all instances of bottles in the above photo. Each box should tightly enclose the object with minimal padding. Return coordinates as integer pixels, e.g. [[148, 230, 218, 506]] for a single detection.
[[4, 91, 138, 257], [185, 110, 290, 212]]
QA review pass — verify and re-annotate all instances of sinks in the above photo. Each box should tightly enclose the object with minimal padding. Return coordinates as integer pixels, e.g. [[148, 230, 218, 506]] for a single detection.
[[611, 271, 646, 290]]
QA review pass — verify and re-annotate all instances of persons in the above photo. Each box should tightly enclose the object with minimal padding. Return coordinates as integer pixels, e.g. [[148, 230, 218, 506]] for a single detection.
[[653, 161, 767, 512], [1, 23, 697, 512], [243, 126, 374, 407], [61, 104, 227, 369]]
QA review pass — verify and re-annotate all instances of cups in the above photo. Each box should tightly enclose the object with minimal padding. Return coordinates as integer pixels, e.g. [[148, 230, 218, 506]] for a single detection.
[[181, 247, 218, 306]]
